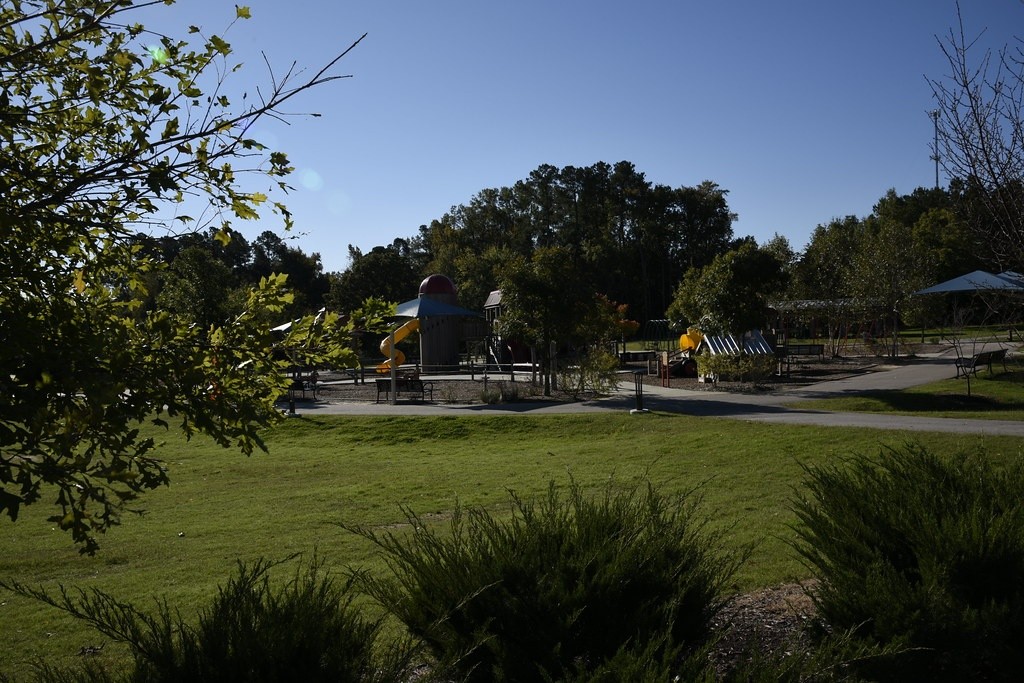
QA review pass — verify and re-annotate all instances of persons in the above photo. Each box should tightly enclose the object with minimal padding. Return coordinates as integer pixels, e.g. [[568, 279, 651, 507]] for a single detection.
[[310, 370, 320, 402]]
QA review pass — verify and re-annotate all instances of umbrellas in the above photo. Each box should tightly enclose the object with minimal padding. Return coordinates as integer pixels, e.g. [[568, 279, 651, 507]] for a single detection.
[[911, 270, 1024, 294], [974, 270, 1024, 299], [361, 295, 483, 371]]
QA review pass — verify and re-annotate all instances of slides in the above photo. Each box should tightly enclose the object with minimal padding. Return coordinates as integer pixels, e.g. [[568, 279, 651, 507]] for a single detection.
[[377, 319, 419, 373]]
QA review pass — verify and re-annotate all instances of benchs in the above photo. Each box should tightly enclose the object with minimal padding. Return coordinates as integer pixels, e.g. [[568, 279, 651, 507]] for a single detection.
[[376, 378, 434, 404], [956, 349, 1008, 379], [785, 344, 825, 361], [291, 379, 320, 402], [620, 352, 656, 368]]
